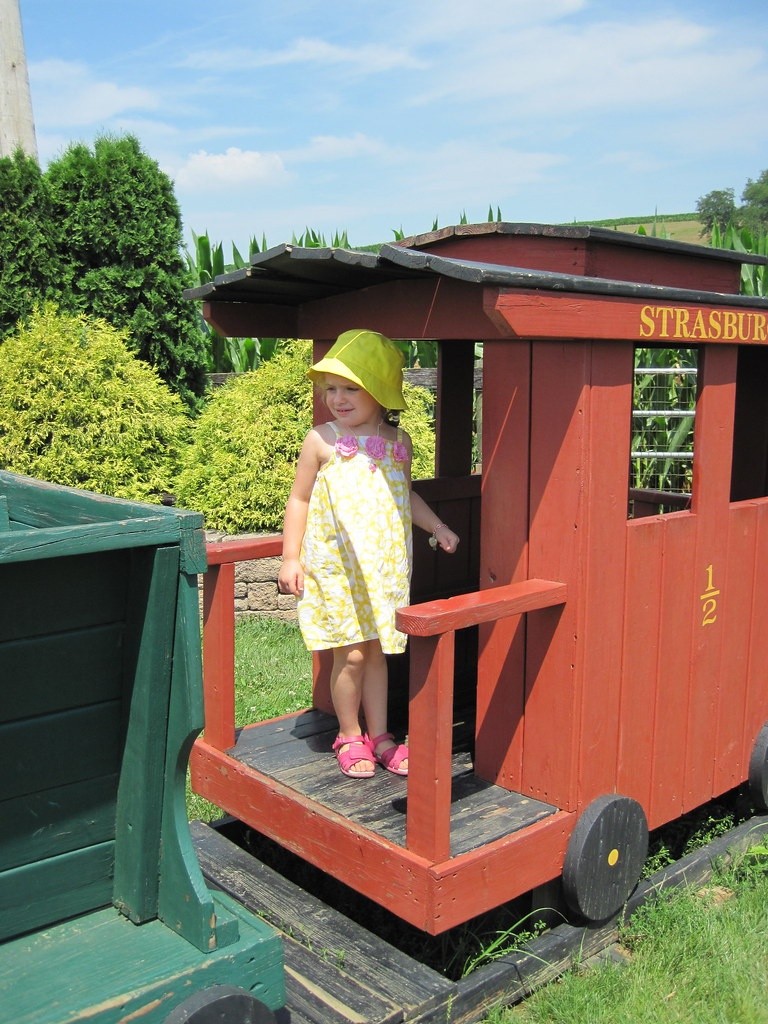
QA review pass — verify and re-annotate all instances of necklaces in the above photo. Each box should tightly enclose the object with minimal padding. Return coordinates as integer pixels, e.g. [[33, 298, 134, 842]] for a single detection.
[[348, 419, 383, 472]]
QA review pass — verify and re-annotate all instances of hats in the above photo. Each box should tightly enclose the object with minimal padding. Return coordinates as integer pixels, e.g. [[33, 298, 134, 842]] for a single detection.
[[306, 328, 409, 411]]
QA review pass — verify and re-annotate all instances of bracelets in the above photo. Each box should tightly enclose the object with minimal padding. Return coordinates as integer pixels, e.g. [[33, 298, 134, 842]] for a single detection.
[[429, 524, 448, 551]]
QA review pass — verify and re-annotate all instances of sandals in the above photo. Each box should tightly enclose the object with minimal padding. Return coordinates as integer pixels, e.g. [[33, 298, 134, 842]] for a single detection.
[[333, 733, 377, 778], [364, 730, 410, 776]]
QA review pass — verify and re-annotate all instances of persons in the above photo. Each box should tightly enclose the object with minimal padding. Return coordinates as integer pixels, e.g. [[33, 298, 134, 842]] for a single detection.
[[278, 329, 460, 778]]
